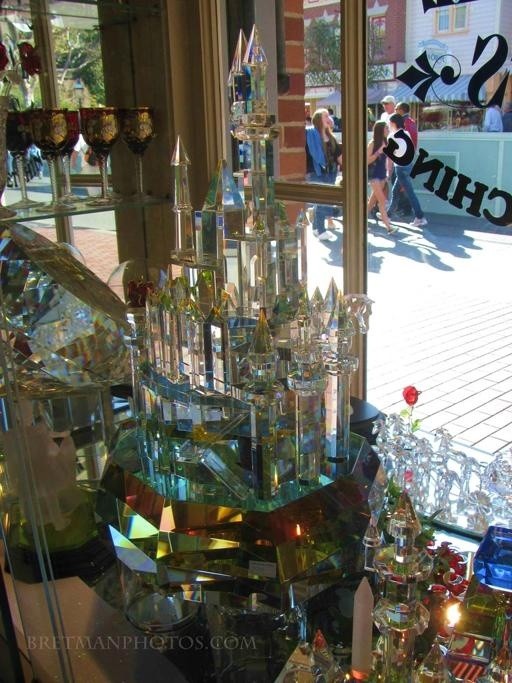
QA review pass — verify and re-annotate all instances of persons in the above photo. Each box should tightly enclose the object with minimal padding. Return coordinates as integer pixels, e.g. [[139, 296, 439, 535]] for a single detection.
[[501, 100, 512, 132], [305, 94, 428, 241], [484, 106, 503, 132]]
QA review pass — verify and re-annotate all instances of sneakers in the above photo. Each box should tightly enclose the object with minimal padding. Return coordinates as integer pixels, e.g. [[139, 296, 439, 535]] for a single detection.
[[308, 207, 336, 240], [375, 211, 428, 235]]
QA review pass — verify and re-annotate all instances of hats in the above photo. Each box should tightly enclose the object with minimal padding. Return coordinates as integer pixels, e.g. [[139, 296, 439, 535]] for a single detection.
[[381, 96, 394, 104]]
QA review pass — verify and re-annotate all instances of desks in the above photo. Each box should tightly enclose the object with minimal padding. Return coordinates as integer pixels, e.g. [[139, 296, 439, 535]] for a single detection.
[[93, 425, 389, 612], [350, 395, 386, 443]]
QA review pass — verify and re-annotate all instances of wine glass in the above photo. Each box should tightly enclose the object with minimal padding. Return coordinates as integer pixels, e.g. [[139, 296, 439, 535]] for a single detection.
[[4, 106, 161, 210]]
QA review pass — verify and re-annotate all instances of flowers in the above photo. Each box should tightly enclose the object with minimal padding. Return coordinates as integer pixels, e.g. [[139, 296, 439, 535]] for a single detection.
[[401, 384, 421, 432]]
